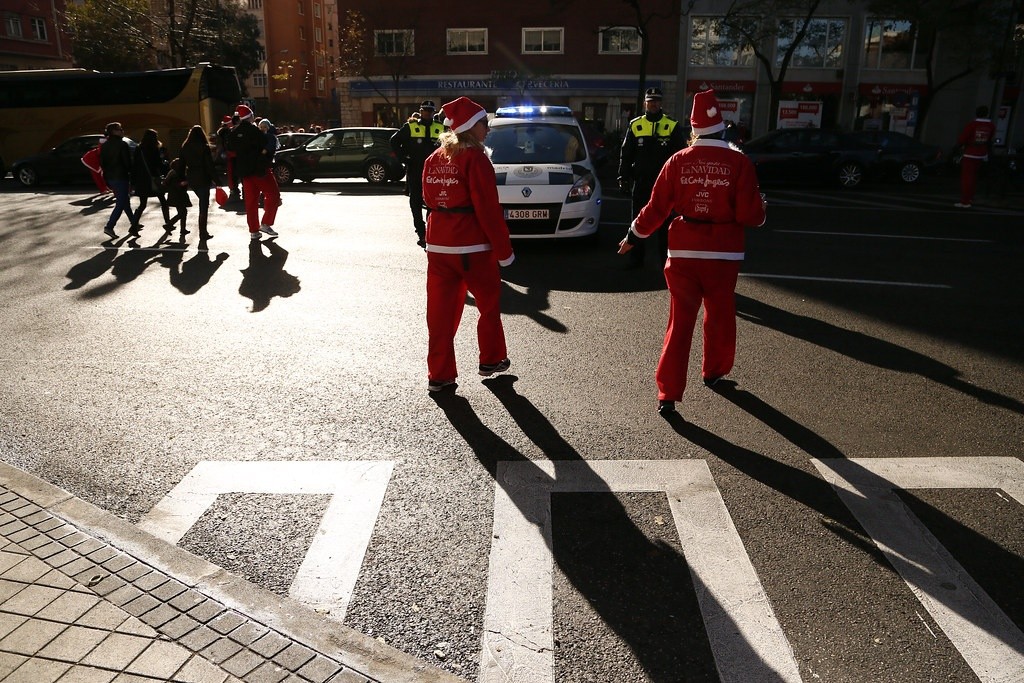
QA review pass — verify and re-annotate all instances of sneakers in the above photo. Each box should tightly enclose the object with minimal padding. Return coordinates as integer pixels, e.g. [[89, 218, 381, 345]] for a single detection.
[[429, 380, 455, 392], [478, 358, 510, 375]]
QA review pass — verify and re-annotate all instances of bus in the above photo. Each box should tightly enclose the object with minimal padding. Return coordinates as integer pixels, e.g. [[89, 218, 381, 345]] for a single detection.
[[0, 62, 246, 180]]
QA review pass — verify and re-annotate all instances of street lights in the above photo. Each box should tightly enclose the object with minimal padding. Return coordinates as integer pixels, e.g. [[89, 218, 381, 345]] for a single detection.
[[263, 49, 289, 97]]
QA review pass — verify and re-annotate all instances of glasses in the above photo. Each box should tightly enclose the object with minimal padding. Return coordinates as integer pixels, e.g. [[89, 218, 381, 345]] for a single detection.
[[478, 119, 489, 127], [422, 108, 433, 112], [114, 128, 123, 131]]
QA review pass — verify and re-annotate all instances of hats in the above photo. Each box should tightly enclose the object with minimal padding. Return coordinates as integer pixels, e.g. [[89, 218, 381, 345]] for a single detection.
[[221, 115, 232, 124], [421, 101, 434, 108], [234, 105, 253, 120], [690, 89, 725, 135], [645, 87, 663, 102], [443, 98, 487, 135]]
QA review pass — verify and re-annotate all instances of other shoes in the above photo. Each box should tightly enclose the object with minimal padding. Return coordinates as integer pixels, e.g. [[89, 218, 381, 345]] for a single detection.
[[417, 229, 426, 247], [171, 226, 177, 230], [128, 228, 141, 237], [954, 202, 971, 208], [104, 228, 118, 238], [181, 229, 190, 234], [657, 400, 675, 413], [200, 231, 214, 239], [703, 375, 723, 385], [130, 222, 145, 228], [259, 224, 278, 235], [162, 224, 172, 236], [251, 231, 262, 240]]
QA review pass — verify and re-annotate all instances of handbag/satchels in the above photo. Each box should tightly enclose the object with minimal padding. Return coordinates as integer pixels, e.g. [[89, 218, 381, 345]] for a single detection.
[[216, 186, 228, 205], [150, 177, 159, 192]]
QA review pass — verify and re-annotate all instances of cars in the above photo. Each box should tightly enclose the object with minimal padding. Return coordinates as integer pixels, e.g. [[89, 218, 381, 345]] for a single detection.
[[857, 130, 945, 185], [481, 105, 602, 248], [739, 125, 884, 193], [12, 134, 139, 188], [272, 126, 406, 188], [278, 132, 317, 151]]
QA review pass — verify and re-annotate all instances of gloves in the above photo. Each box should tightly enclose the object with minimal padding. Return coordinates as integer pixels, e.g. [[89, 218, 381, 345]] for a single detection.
[[618, 176, 628, 193]]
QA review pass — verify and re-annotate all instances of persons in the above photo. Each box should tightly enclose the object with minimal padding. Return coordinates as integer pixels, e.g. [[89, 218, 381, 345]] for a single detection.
[[618, 89, 681, 268], [618, 89, 766, 413], [957, 106, 996, 208], [81, 104, 324, 241], [421, 96, 515, 393], [377, 101, 444, 248]]
